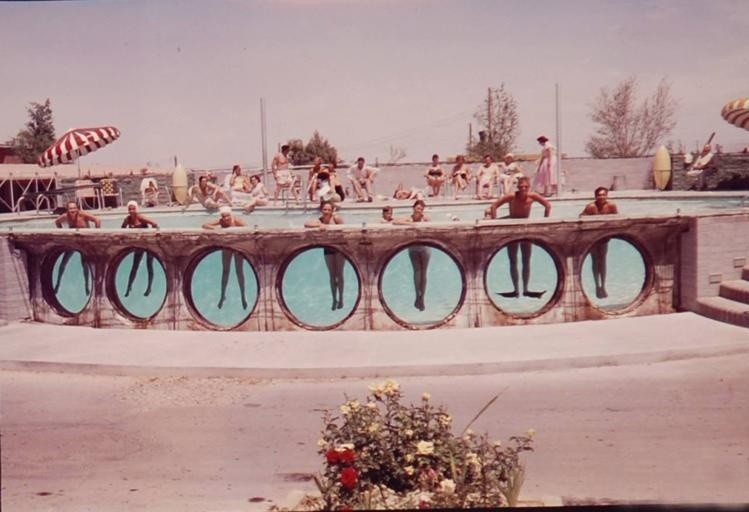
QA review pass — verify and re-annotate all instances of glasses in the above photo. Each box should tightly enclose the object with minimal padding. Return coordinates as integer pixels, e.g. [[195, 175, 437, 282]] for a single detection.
[[222, 214, 231, 218], [128, 208, 136, 211]]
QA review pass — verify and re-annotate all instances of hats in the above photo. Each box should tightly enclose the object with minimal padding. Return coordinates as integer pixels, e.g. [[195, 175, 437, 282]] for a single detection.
[[127, 200, 137, 208], [537, 135, 549, 143], [220, 205, 232, 214], [505, 153, 513, 158]]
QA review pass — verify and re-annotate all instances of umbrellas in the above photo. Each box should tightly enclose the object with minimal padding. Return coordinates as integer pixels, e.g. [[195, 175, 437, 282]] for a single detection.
[[38, 126, 121, 180], [722, 100, 749, 131]]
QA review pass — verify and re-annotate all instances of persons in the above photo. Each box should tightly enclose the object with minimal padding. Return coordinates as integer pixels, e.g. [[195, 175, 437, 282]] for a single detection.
[[687, 143, 718, 191], [578, 186, 618, 299], [53, 201, 101, 294]]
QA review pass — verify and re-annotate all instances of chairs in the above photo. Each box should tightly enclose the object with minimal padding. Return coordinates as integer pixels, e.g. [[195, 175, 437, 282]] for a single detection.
[[98, 175, 125, 209], [428, 170, 520, 200], [279, 173, 302, 204], [139, 176, 162, 207], [74, 178, 102, 212], [223, 173, 251, 202]]
